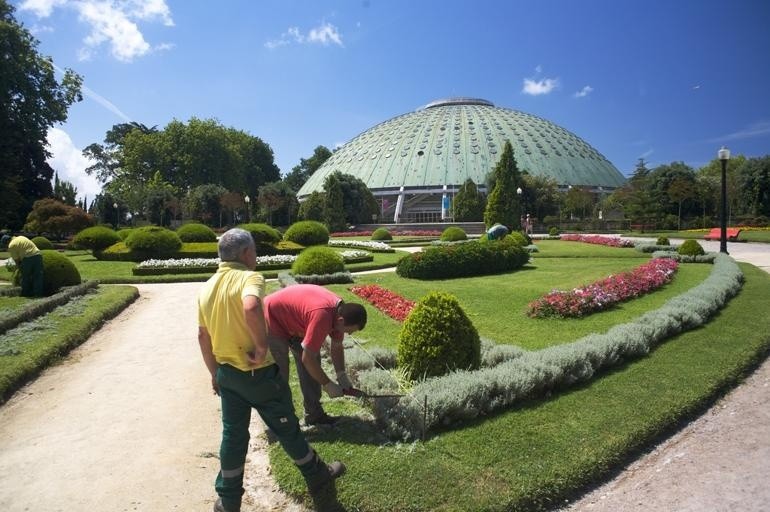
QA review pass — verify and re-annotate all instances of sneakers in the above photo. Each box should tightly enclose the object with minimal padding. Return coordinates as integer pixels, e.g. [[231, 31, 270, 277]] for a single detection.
[[213, 496, 241, 512], [310, 461, 345, 487], [305, 413, 335, 424]]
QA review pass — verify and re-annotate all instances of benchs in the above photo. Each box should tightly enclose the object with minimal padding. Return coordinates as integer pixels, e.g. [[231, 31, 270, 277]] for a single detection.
[[703, 228, 745, 241]]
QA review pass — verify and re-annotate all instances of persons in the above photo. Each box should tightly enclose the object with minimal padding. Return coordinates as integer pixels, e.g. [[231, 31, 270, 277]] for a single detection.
[[264, 284, 367, 426], [196, 228, 344, 512], [1, 235, 43, 296], [521, 213, 533, 234]]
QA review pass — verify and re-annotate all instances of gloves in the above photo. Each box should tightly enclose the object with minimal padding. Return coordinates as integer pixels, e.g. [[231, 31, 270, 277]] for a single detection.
[[323, 372, 352, 397]]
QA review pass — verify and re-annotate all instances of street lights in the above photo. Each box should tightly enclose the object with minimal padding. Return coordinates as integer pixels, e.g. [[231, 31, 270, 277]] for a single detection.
[[112, 203, 119, 231], [244, 194, 251, 224], [516, 186, 524, 231], [717, 144, 731, 254]]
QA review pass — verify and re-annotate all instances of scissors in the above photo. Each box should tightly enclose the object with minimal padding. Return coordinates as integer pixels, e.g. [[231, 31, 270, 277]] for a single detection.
[[342, 386, 406, 399]]
